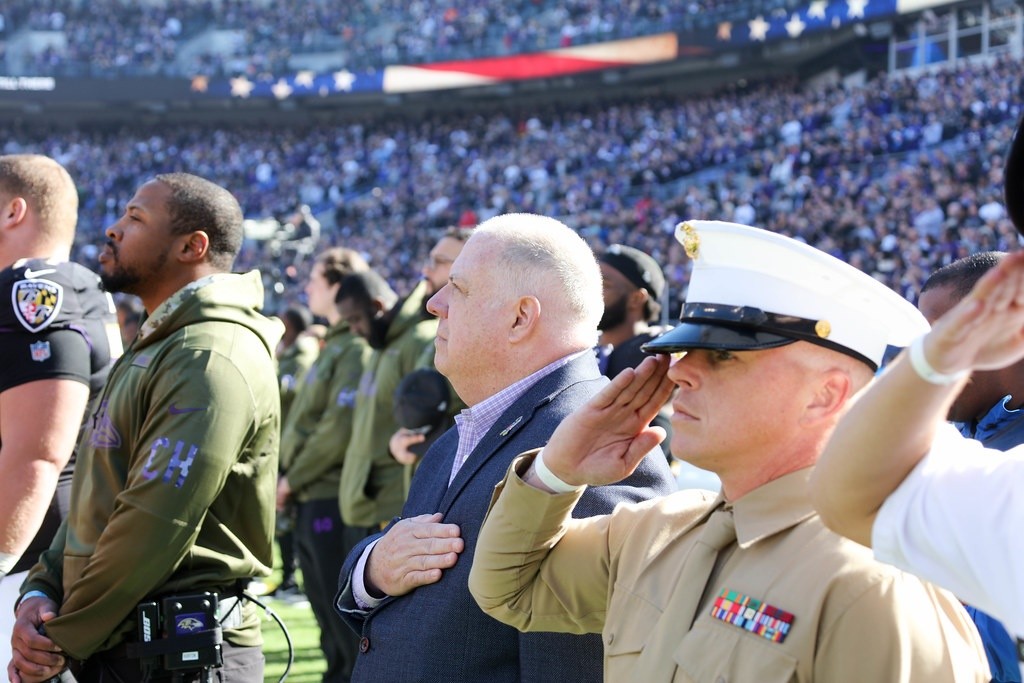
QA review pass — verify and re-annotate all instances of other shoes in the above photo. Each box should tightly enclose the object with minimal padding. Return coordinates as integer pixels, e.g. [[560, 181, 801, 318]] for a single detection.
[[268, 583, 308, 602]]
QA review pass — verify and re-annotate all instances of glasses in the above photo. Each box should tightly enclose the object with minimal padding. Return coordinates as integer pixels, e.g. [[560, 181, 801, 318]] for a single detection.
[[426, 257, 454, 269]]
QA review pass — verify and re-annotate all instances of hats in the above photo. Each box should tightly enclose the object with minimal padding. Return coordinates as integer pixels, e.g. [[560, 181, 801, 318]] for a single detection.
[[393, 369, 448, 457], [1004, 112, 1024, 237], [640, 219, 932, 373], [595, 244, 664, 304]]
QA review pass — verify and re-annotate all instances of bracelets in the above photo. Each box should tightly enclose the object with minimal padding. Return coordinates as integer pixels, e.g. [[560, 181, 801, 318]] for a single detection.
[[535, 450, 588, 493], [911, 335, 970, 383], [0, 553, 20, 575], [16, 590, 48, 611]]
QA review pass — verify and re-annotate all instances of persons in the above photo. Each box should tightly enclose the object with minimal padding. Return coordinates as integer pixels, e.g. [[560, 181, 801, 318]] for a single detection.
[[333, 212, 677, 683], [6, 172, 285, 683], [593, 243, 683, 463], [273, 232, 469, 683], [0, 154, 125, 683], [1, 1, 1024, 323], [464, 220, 992, 683], [913, 252, 1024, 683], [811, 126, 1023, 640]]
